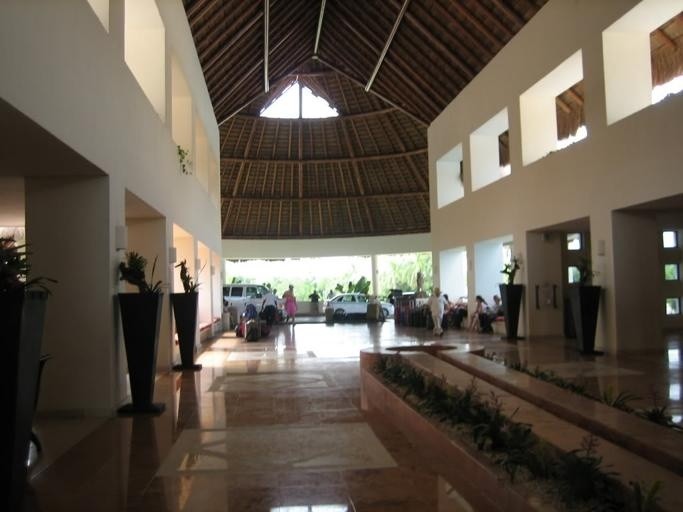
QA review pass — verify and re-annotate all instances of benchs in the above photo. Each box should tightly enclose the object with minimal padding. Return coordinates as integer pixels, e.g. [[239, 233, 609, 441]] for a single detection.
[[175, 318, 221, 357], [491, 316, 505, 335]]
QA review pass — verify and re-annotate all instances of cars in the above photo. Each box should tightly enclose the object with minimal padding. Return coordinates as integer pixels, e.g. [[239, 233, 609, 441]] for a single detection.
[[325, 293, 391, 321]]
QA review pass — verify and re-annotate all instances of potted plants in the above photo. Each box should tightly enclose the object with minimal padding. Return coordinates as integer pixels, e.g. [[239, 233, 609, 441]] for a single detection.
[[567, 253, 601, 355], [169, 261, 203, 371], [115, 249, 166, 416], [0, 236, 59, 511], [499, 256, 525, 340], [363, 351, 682, 511]]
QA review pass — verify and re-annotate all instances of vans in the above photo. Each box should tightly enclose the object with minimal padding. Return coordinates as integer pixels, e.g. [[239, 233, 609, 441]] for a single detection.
[[223, 284, 276, 318]]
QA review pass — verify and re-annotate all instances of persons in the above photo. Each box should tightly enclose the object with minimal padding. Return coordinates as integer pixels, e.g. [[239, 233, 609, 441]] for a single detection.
[[259, 287, 281, 330], [467, 296, 488, 330], [451, 308, 467, 330], [442, 293, 449, 329], [309, 290, 321, 316], [283, 325, 296, 369], [282, 294, 297, 328], [372, 295, 386, 322], [428, 287, 450, 337], [282, 284, 294, 298], [327, 290, 336, 300], [273, 289, 278, 309], [478, 295, 503, 333]]
